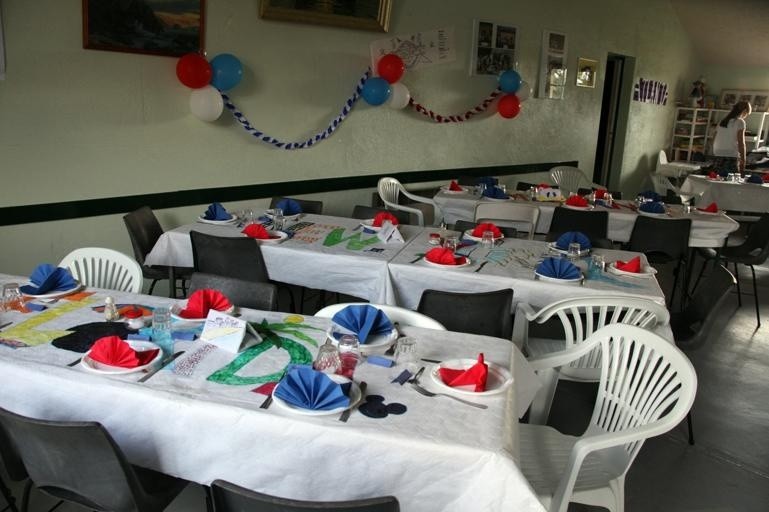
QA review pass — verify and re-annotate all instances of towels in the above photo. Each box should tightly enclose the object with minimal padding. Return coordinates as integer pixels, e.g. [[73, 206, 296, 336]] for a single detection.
[[449, 180, 463, 191], [274, 365, 352, 410], [696, 202, 717, 213], [178, 288, 233, 319], [639, 201, 665, 213], [199, 201, 233, 221], [761, 172, 769, 183], [533, 183, 550, 192], [240, 224, 281, 239], [19, 262, 80, 295], [482, 185, 510, 199], [535, 256, 581, 280], [556, 231, 592, 251], [438, 352, 489, 393], [593, 189, 621, 210], [475, 177, 497, 185], [636, 190, 661, 202], [424, 246, 466, 265], [87, 335, 160, 368], [275, 198, 304, 216], [614, 255, 641, 273], [471, 223, 502, 238], [707, 171, 717, 179], [329, 304, 394, 344], [565, 194, 588, 207], [372, 211, 400, 227], [745, 174, 764, 184]]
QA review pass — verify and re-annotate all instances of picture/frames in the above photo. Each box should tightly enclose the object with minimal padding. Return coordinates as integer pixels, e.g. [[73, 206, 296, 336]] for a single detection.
[[718, 89, 769, 112], [536, 27, 569, 100], [575, 57, 599, 89], [467, 17, 521, 78], [258, 0, 393, 34], [82, 0, 205, 59]]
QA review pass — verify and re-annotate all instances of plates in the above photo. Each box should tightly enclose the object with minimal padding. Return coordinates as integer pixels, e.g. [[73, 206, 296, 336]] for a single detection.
[[81, 338, 163, 375], [609, 260, 658, 280], [322, 321, 400, 349], [168, 296, 234, 323], [358, 217, 395, 233], [440, 184, 727, 217], [260, 209, 301, 222], [429, 360, 515, 396], [273, 371, 363, 416], [253, 229, 290, 246], [16, 280, 81, 298], [422, 251, 468, 271], [546, 240, 590, 259], [532, 267, 585, 283], [462, 228, 505, 243], [197, 210, 239, 226]]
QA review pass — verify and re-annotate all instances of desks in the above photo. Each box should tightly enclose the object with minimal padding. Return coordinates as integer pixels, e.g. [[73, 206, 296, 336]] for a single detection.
[[432, 186, 741, 249], [143, 210, 425, 305], [679, 173, 769, 216], [386, 228, 667, 323], [0, 273, 550, 512]]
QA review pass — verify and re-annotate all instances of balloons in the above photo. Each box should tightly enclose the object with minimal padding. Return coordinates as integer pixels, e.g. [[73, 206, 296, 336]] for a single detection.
[[189, 85, 225, 122], [516, 81, 529, 101], [362, 77, 390, 108], [496, 95, 520, 120], [211, 51, 242, 92], [177, 53, 212, 88], [499, 70, 521, 93], [390, 84, 409, 110], [378, 51, 404, 85]]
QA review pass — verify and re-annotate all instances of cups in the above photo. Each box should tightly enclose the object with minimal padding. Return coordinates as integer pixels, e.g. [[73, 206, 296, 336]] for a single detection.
[[479, 231, 496, 250], [734, 173, 741, 182], [726, 173, 734, 182], [567, 242, 582, 261], [0, 283, 25, 312]]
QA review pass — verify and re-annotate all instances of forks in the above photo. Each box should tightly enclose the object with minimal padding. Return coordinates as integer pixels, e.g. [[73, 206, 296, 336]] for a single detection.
[[411, 384, 489, 411]]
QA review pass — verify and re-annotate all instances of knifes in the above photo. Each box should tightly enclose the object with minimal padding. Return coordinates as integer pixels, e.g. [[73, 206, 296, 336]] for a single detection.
[[472, 258, 489, 274], [138, 349, 185, 384]]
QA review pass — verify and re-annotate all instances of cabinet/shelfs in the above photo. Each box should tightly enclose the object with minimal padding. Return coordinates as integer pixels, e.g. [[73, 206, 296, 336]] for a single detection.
[[704, 109, 769, 153], [668, 106, 712, 162]]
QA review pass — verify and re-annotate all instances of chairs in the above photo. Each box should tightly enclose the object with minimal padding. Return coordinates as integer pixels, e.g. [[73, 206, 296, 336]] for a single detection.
[[546, 233, 613, 250], [669, 263, 737, 445], [691, 211, 767, 328], [454, 219, 517, 238], [0, 407, 191, 512], [313, 302, 449, 331], [210, 479, 399, 512], [122, 205, 194, 298], [55, 247, 143, 294], [547, 165, 607, 192], [269, 194, 323, 216], [377, 177, 438, 227], [508, 322, 698, 512], [351, 205, 410, 225], [474, 202, 540, 241], [187, 270, 279, 311], [546, 206, 608, 237], [648, 171, 700, 206], [513, 292, 672, 424], [415, 287, 514, 338], [620, 214, 692, 308], [188, 230, 272, 283]]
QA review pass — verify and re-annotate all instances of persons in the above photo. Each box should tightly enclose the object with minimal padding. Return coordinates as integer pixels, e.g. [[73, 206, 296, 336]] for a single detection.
[[712, 101, 752, 175]]
